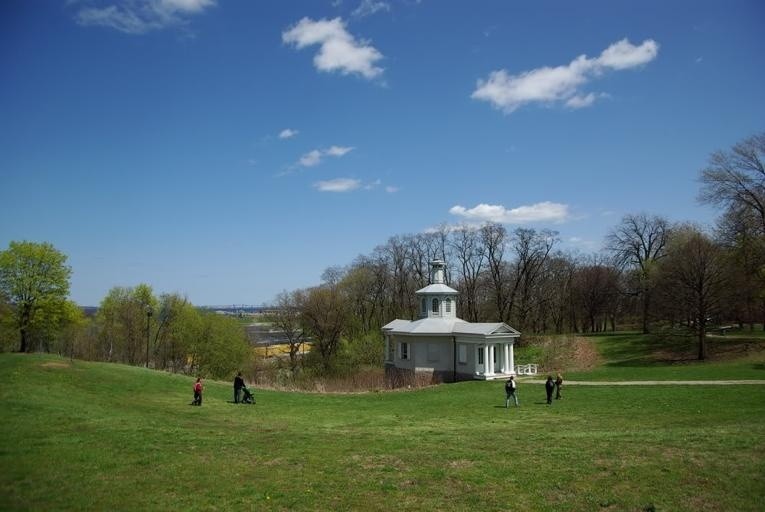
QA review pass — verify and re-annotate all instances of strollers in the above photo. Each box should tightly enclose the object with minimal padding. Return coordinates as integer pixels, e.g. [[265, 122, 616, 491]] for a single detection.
[[241, 387, 256, 404]]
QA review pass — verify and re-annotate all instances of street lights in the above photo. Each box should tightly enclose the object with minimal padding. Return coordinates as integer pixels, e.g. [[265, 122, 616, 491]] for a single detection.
[[144, 304, 153, 368]]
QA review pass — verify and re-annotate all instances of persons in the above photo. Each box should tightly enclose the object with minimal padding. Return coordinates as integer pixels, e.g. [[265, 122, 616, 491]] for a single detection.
[[545, 375, 555, 405], [505, 375, 520, 408], [233, 371, 245, 402], [193, 377, 204, 406], [555, 373, 563, 401]]
